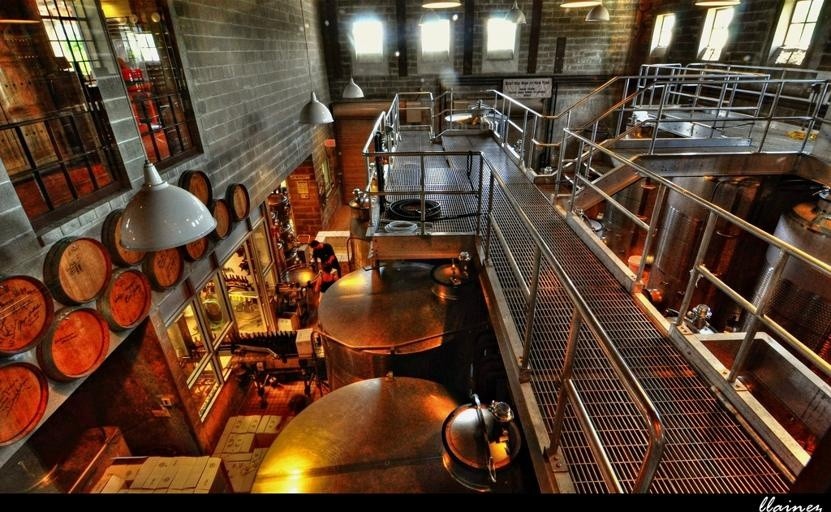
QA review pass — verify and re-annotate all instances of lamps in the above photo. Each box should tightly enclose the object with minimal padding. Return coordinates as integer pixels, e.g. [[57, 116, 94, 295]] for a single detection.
[[585, 0, 610, 22], [503, 1, 526, 25], [298, 0, 365, 124], [92, 0, 219, 252]]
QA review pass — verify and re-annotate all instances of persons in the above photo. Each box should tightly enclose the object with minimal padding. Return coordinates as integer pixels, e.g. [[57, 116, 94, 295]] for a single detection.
[[322, 262, 340, 283], [116, 55, 152, 127], [309, 239, 342, 279]]
